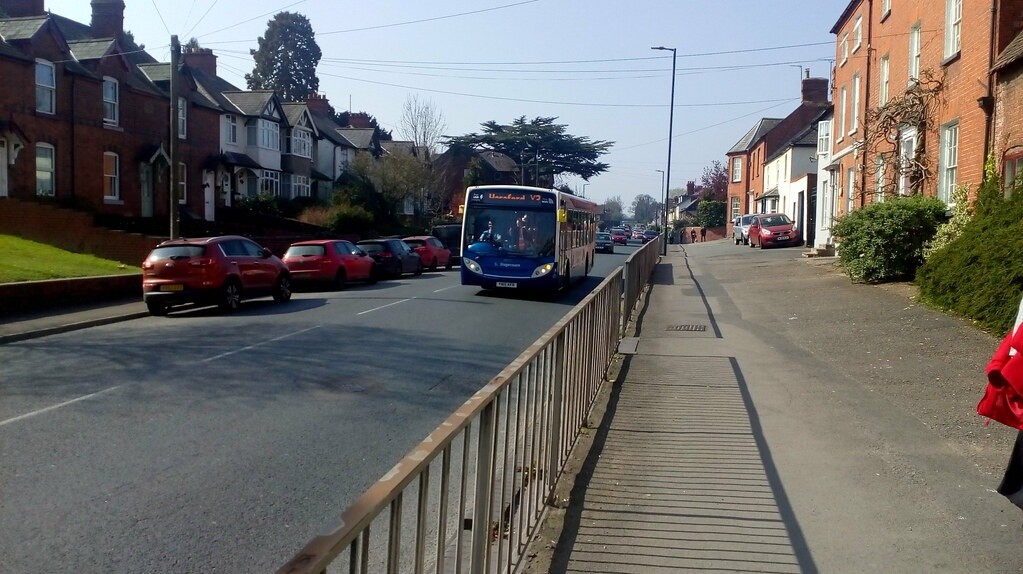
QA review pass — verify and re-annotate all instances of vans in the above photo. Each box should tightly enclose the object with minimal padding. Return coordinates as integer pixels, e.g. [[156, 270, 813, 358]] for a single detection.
[[431, 224, 463, 261]]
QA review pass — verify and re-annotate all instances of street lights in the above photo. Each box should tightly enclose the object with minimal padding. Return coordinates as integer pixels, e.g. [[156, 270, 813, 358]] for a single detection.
[[650, 45, 677, 255], [656, 169, 665, 232]]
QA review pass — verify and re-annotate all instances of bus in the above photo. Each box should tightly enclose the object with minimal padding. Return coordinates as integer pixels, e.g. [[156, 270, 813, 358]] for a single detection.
[[459, 183, 598, 295]]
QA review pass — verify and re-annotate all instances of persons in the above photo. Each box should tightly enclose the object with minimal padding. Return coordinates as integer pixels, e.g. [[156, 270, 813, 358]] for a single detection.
[[679, 228, 685, 244], [478, 211, 545, 254], [668, 229, 675, 244], [690, 228, 697, 243], [996, 290, 1023, 512], [700, 225, 707, 242]]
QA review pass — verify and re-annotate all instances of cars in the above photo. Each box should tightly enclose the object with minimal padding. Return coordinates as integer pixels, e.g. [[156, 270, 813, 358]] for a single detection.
[[732, 214, 759, 245], [594, 232, 614, 253], [355, 237, 423, 280], [401, 235, 453, 272], [642, 230, 658, 244], [610, 227, 643, 245], [141, 235, 294, 317], [281, 239, 381, 292], [748, 213, 801, 249]]
